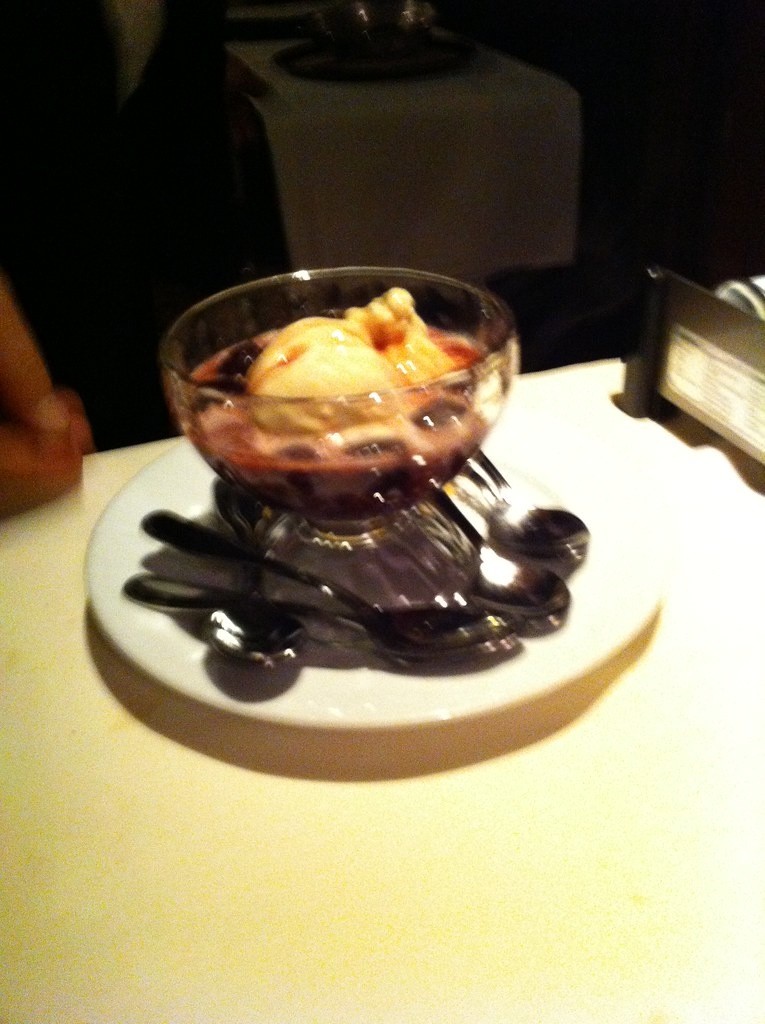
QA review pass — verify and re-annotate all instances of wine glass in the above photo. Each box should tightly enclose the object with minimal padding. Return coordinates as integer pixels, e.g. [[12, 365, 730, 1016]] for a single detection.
[[162, 267, 521, 607]]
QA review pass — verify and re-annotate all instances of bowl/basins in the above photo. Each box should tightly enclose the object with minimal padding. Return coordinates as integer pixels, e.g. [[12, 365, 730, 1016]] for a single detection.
[[289, 0, 435, 63]]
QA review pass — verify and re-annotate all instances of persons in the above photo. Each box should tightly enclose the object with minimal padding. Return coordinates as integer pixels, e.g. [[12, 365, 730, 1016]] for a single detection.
[[0, 0, 283, 512]]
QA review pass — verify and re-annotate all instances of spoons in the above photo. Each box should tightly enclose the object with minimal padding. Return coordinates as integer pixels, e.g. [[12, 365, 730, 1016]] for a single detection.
[[126, 423, 593, 675]]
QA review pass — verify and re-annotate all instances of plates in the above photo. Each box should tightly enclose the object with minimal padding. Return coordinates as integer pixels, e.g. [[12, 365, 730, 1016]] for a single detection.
[[273, 41, 463, 82], [86, 423, 664, 735]]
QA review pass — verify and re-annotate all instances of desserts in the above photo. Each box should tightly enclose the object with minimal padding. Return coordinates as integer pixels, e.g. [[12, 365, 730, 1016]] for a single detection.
[[156, 264, 519, 536]]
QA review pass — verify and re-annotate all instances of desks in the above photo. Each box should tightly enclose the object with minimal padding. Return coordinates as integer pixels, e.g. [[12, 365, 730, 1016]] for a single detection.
[[0, 358, 765, 1024]]
